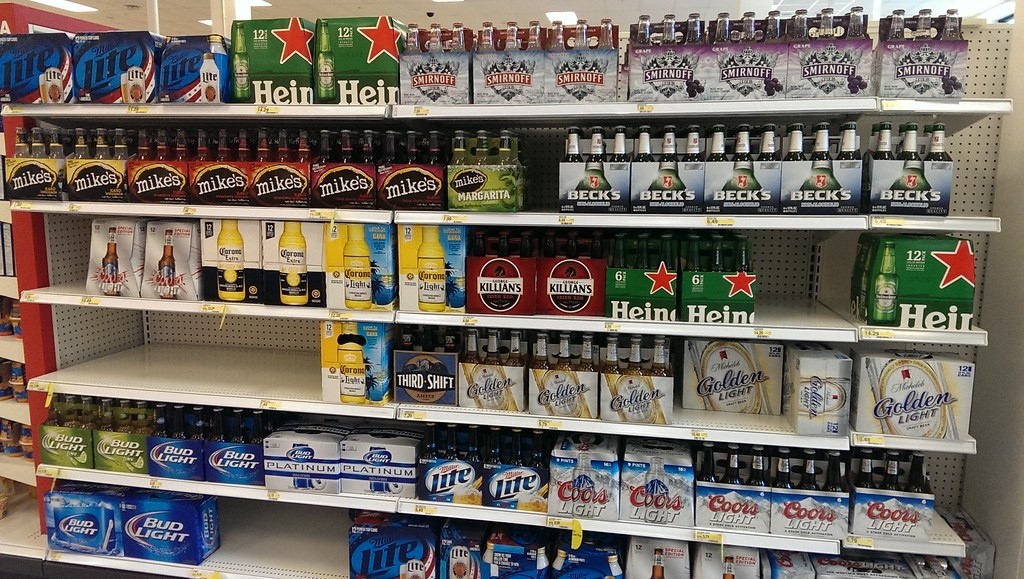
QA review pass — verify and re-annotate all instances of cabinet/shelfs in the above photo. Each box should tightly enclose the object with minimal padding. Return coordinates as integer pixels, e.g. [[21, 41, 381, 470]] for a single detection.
[[0, 132, 49, 579], [0, 97, 1014, 579]]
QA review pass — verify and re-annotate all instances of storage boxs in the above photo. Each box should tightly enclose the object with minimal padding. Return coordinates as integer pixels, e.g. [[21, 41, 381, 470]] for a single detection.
[[0, 15, 969, 105], [348, 511, 447, 579], [44, 481, 135, 557], [439, 519, 554, 579], [759, 548, 816, 579], [901, 553, 969, 579], [692, 543, 761, 579], [932, 505, 996, 579], [5, 138, 955, 217], [808, 545, 917, 579], [624, 536, 691, 579], [85, 219, 976, 331], [118, 487, 220, 565], [549, 529, 624, 579], [321, 319, 976, 440], [39, 402, 935, 545]]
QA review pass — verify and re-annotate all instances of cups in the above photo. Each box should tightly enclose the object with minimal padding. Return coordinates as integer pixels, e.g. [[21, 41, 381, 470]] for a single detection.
[[121, 73, 128, 104], [400, 565, 406, 579], [39, 74, 45, 102]]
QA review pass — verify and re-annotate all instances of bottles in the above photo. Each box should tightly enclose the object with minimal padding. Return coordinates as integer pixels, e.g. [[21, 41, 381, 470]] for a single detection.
[[537, 546, 549, 569], [562, 122, 953, 163], [608, 555, 623, 576], [716, 161, 763, 215], [232, 22, 251, 100], [796, 161, 843, 215], [316, 20, 336, 102], [650, 548, 666, 579], [102, 227, 119, 296], [278, 222, 308, 305], [13, 126, 514, 165], [44, 395, 283, 445], [200, 35, 229, 103], [572, 163, 613, 212], [406, 4, 964, 55], [417, 227, 446, 312], [482, 541, 494, 564], [421, 422, 547, 469], [552, 549, 567, 570], [400, 325, 668, 377], [466, 230, 750, 272], [698, 442, 928, 494], [723, 556, 735, 579], [336, 322, 370, 404], [886, 160, 932, 215], [870, 241, 900, 324], [158, 230, 175, 299], [217, 219, 245, 301], [343, 224, 372, 310], [646, 162, 688, 213]]
